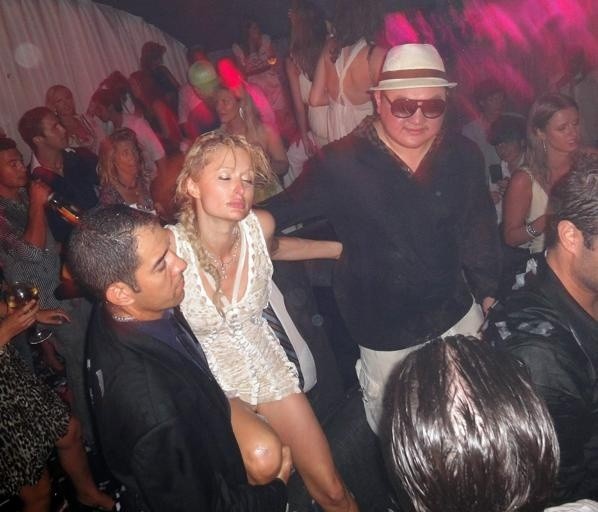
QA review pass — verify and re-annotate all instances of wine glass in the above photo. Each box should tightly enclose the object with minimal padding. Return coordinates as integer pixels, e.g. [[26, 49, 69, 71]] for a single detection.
[[3, 279, 53, 345]]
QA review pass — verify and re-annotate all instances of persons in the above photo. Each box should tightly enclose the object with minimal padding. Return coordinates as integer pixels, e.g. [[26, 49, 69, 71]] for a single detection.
[[63, 202, 294, 512], [381, 333, 595, 512], [0, 86, 166, 510], [255, 44, 496, 436], [478, 157, 597, 504], [98, 0, 597, 394], [160, 129, 359, 512]]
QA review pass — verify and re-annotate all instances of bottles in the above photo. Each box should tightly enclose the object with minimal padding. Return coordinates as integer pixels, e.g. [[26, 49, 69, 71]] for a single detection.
[[44, 191, 84, 228]]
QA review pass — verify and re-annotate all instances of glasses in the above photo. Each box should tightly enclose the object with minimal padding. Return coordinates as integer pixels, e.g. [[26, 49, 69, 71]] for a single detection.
[[383, 91, 448, 118]]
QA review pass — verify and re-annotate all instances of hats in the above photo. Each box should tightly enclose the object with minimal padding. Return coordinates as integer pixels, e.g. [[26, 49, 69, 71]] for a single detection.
[[367, 43, 458, 91]]
